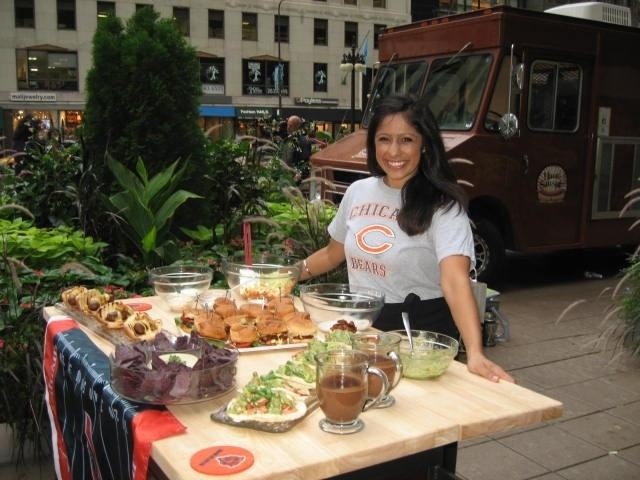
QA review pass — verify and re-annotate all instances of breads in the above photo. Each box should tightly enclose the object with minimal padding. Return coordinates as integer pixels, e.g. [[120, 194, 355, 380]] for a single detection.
[[62, 284, 162, 341]]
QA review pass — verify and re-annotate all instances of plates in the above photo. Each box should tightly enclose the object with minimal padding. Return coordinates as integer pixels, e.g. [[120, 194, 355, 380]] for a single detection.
[[211, 387, 320, 433], [55, 301, 172, 348], [172, 315, 307, 353]]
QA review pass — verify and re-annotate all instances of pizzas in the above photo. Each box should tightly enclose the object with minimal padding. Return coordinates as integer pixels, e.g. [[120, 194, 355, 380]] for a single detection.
[[226, 342, 352, 423]]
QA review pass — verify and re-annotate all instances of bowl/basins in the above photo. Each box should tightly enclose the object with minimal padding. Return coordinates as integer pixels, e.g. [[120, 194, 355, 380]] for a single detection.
[[220, 255, 304, 298], [384, 329, 459, 380], [298, 281, 385, 330], [146, 262, 214, 306]]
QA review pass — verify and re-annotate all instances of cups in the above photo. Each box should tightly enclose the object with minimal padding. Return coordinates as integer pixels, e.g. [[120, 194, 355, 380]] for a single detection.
[[352, 332, 403, 407], [314, 348, 388, 434]]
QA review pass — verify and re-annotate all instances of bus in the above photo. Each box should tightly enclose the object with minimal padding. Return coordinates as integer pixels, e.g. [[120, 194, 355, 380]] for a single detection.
[[309, 2, 639, 289]]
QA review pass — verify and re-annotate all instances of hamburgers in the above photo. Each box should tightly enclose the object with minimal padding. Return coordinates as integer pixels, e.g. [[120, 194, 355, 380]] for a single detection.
[[181, 296, 318, 348]]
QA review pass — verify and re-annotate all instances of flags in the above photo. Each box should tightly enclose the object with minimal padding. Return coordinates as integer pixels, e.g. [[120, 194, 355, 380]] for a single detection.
[[360, 38, 368, 76]]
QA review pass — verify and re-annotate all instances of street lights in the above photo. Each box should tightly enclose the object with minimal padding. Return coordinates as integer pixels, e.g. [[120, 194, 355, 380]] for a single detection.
[[337, 41, 367, 135]]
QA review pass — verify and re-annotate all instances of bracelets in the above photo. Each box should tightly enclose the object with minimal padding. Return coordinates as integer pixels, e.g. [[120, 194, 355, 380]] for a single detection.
[[303, 259, 314, 279]]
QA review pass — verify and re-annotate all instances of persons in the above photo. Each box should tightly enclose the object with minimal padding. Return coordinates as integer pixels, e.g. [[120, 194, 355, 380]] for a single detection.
[[9, 111, 57, 229], [270, 92, 517, 480], [276, 115, 312, 188]]
